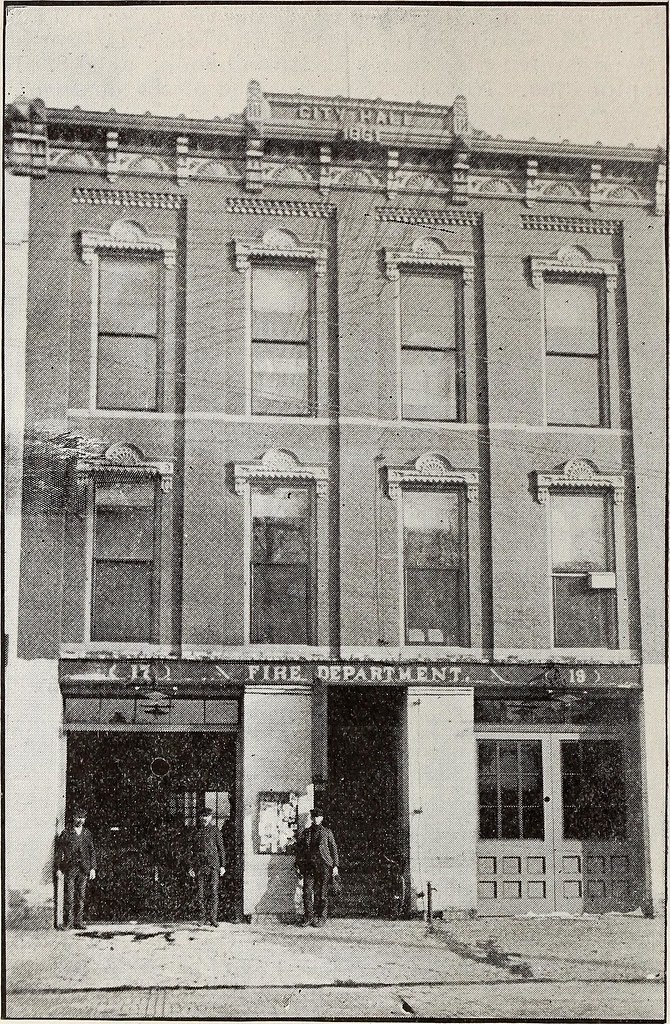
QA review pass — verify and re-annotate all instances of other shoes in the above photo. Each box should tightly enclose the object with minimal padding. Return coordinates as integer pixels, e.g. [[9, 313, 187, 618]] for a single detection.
[[197, 916, 206, 926], [210, 917, 220, 928], [73, 922, 85, 928], [60, 922, 74, 930]]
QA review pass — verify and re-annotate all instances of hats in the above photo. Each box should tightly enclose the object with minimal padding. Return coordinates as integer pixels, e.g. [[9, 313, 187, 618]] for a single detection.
[[310, 808, 323, 817], [196, 807, 212, 816], [72, 808, 87, 818]]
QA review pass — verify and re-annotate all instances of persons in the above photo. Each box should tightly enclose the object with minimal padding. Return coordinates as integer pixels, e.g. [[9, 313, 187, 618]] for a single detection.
[[294, 808, 339, 927], [185, 809, 226, 928], [55, 809, 97, 930]]
